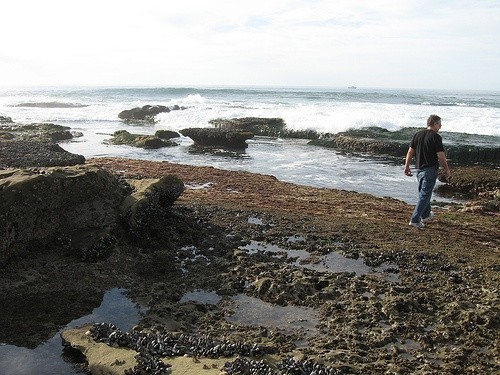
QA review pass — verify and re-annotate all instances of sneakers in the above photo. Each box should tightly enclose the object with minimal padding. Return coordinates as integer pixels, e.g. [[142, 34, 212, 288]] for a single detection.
[[421, 211, 434, 222], [409, 220, 425, 227]]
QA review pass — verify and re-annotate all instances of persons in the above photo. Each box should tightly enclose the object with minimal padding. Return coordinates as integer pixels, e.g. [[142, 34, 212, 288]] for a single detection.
[[404, 115, 450, 229]]
